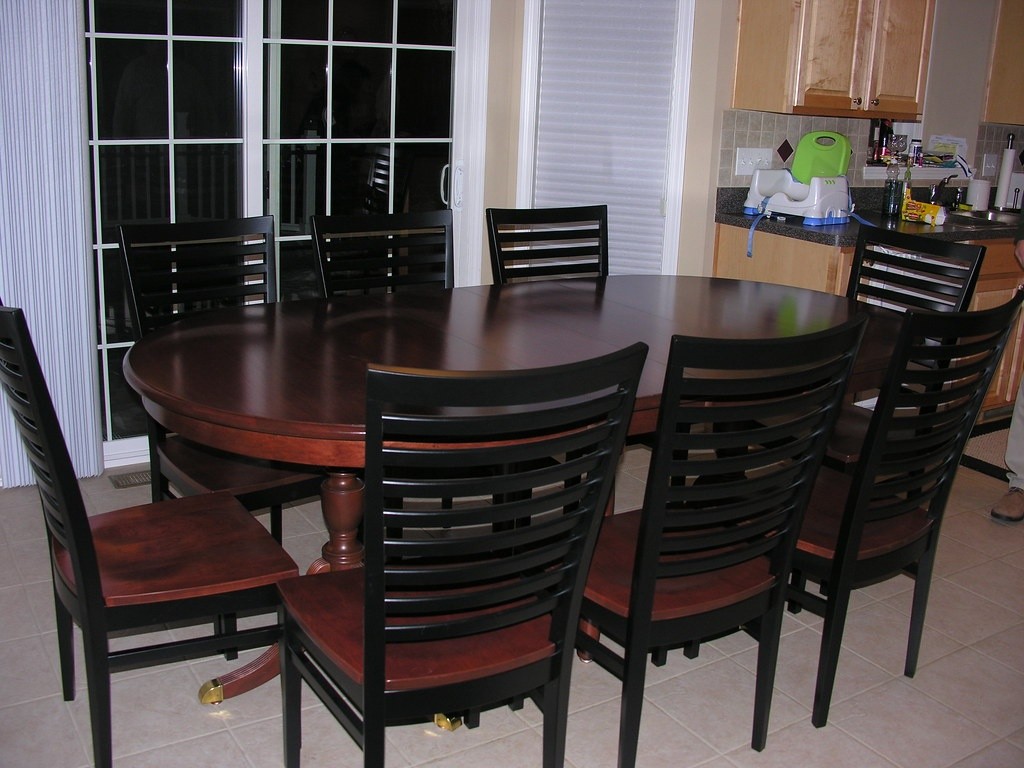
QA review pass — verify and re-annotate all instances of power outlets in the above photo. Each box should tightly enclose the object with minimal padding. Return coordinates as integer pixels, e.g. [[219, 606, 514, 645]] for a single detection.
[[735, 148, 773, 177]]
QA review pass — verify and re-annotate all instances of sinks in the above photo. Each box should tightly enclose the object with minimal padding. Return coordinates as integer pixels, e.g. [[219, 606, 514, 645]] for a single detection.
[[963, 212, 1024, 223], [944, 214, 1008, 227]]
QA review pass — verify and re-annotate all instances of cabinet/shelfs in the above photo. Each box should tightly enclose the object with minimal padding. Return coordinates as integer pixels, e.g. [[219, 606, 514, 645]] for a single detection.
[[713, 222, 1024, 419], [980, 0, 1024, 126], [730, 0, 937, 123]]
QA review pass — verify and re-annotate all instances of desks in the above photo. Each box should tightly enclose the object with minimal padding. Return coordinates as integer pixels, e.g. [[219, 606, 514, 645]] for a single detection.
[[120, 270, 874, 708]]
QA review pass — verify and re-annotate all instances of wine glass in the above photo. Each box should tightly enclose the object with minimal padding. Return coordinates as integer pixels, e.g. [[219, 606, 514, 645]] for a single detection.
[[888, 134, 907, 163]]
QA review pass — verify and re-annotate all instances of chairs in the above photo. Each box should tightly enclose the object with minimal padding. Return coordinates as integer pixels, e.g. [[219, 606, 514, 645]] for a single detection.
[[681, 283, 1024, 727], [113, 214, 324, 659], [310, 204, 455, 544], [267, 339, 651, 768], [0, 297, 302, 768], [576, 304, 875, 768], [483, 204, 611, 528], [781, 220, 990, 609]]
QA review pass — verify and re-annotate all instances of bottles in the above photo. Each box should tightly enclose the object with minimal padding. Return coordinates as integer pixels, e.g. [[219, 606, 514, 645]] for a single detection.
[[881, 137, 887, 156], [919, 153, 923, 166], [873, 141, 878, 160], [955, 186, 964, 209], [880, 157, 901, 221], [908, 153, 914, 166], [901, 164, 912, 220], [909, 139, 922, 167]]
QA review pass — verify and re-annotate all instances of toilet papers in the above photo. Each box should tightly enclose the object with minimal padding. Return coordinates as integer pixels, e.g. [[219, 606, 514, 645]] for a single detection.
[[994, 148, 1016, 208]]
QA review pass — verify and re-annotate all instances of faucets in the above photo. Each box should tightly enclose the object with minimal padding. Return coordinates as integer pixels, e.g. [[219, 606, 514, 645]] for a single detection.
[[938, 179, 948, 206]]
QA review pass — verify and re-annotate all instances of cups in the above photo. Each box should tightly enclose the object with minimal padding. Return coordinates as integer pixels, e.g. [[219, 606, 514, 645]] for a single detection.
[[966, 179, 991, 211]]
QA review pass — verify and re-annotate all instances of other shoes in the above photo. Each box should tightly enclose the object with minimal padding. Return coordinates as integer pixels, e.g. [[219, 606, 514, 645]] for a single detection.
[[990, 486, 1024, 523]]
